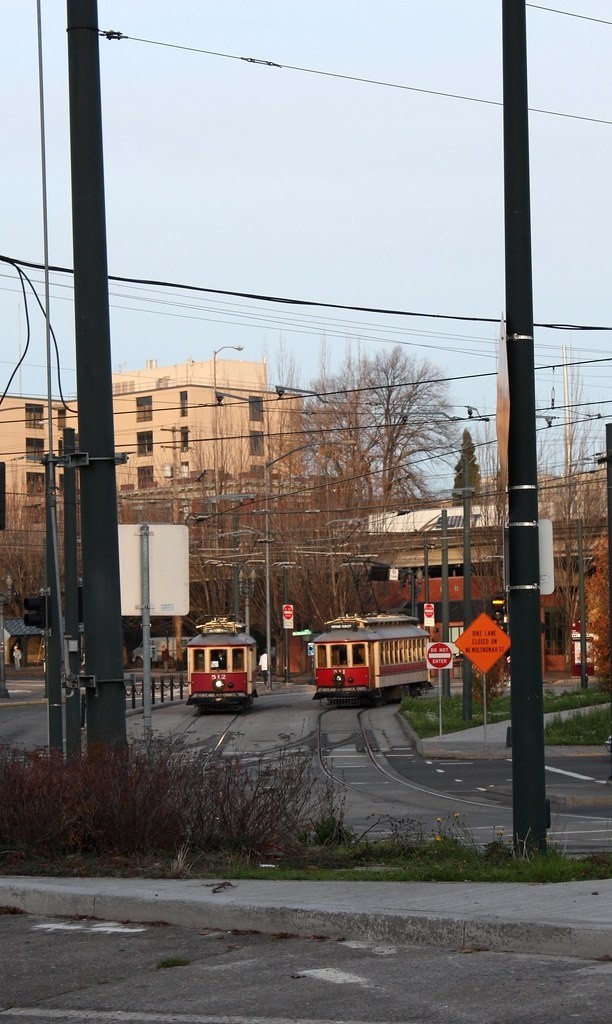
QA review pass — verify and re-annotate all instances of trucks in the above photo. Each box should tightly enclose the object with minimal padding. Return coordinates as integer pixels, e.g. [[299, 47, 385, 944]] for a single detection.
[[132, 635, 194, 668]]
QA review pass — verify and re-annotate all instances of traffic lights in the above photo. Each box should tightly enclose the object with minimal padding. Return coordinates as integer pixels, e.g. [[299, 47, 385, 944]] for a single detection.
[[491, 593, 506, 622], [23, 591, 48, 631]]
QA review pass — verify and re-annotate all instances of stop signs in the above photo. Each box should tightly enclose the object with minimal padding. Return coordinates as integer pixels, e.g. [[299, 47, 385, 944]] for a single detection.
[[283, 605, 293, 620], [426, 642, 455, 671], [423, 604, 434, 619]]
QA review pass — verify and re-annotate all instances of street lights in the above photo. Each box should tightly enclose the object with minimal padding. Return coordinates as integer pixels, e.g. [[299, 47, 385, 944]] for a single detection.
[[212, 342, 248, 535], [264, 439, 359, 688]]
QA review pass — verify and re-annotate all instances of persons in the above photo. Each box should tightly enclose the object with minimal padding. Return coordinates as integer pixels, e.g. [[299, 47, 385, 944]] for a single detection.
[[13, 646, 22, 672], [212, 650, 227, 671], [259, 651, 267, 685], [161, 645, 169, 673]]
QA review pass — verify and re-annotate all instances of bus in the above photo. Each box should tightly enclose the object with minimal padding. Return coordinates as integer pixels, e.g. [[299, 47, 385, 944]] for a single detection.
[[186, 613, 258, 715], [313, 613, 435, 707]]
